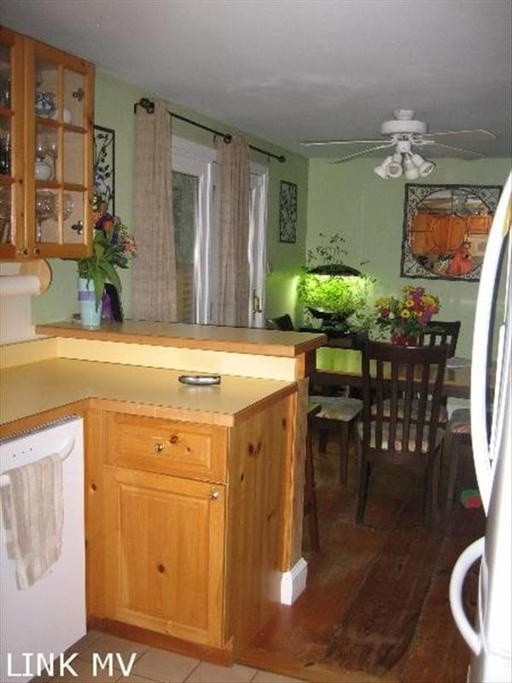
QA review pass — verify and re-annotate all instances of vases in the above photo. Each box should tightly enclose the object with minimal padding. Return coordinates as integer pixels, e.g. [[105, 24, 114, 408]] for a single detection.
[[77, 278, 104, 328]]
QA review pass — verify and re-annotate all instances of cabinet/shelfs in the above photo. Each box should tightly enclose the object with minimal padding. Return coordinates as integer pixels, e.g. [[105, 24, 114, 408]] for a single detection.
[[0, 25, 96, 260], [95, 387, 293, 667]]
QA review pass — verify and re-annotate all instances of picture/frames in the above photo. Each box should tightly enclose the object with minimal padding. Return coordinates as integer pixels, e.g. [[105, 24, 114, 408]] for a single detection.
[[93, 125, 116, 228], [279, 180, 297, 244], [400, 184, 504, 282]]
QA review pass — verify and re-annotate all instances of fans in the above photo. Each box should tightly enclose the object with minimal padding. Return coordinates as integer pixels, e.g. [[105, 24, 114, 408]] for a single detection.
[[298, 109, 499, 164]]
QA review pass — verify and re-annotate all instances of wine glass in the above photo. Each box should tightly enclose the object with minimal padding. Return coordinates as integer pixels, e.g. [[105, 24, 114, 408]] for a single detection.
[[35, 190, 74, 243], [36, 137, 58, 182]]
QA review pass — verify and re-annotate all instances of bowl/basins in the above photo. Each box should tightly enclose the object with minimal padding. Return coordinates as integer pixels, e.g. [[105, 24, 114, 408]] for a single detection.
[[35, 90, 56, 115]]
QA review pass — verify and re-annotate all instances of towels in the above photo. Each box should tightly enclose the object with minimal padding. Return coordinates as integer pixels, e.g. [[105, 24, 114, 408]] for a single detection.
[[0, 453, 64, 590]]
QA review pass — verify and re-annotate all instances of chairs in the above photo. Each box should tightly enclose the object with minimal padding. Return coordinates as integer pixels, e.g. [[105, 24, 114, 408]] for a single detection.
[[269, 315, 493, 552]]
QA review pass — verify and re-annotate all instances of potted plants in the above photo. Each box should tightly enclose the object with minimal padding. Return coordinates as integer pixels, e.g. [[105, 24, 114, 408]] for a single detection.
[[296, 233, 377, 332]]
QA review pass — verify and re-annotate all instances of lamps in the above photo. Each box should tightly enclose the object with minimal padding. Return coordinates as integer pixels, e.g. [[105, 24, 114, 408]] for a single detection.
[[374, 141, 434, 180], [308, 264, 363, 282]]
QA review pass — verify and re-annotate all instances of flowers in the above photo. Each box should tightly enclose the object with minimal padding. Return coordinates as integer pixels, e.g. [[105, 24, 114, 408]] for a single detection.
[[71, 138, 139, 314], [375, 284, 442, 336]]
